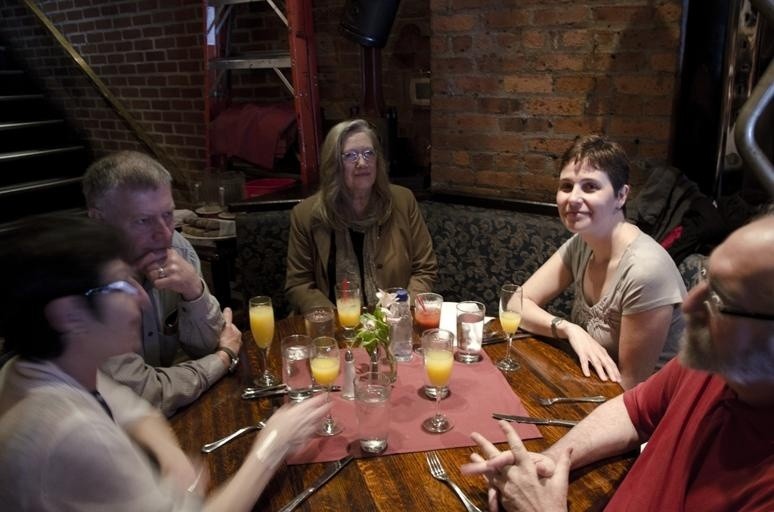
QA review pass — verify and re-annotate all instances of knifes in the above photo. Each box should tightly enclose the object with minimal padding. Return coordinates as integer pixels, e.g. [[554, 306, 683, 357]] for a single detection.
[[483, 334, 534, 344], [492, 413, 579, 426], [242, 383, 289, 398]]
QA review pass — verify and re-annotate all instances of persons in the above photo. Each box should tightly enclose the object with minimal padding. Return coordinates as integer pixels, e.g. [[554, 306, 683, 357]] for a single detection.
[[75, 150, 243, 422], [0, 213, 336, 511], [459, 213, 773, 511], [284, 120, 440, 311], [506, 135, 689, 394]]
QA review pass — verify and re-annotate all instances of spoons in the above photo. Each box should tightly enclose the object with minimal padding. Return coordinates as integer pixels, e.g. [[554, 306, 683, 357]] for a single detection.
[[275, 439, 387, 512]]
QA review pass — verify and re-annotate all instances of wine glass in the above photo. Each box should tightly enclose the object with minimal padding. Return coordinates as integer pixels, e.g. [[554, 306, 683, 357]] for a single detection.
[[281, 281, 523, 434], [249, 296, 280, 387]]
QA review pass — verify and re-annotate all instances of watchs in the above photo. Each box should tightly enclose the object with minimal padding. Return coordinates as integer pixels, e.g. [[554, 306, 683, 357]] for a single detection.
[[216, 347, 240, 374], [551, 316, 564, 341]]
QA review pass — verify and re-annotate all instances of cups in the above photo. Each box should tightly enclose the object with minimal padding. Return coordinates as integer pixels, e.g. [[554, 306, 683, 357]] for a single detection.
[[351, 368, 390, 456]]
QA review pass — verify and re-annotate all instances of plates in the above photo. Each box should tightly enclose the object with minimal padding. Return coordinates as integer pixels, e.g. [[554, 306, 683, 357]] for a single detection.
[[174, 206, 237, 239]]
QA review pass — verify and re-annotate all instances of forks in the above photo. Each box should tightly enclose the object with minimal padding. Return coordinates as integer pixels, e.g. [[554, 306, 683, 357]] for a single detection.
[[530, 392, 607, 406], [425, 452, 482, 512], [202, 416, 268, 452]]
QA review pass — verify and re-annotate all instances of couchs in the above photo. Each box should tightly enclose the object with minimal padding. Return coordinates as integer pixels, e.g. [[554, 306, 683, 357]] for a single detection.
[[232, 201, 712, 375]]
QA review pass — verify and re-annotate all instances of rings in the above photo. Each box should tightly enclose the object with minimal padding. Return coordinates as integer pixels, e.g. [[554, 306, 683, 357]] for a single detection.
[[159, 267, 165, 278]]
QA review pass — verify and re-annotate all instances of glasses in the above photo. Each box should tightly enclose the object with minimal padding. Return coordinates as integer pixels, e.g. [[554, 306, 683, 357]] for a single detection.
[[699, 260, 774, 321], [342, 150, 376, 162]]
